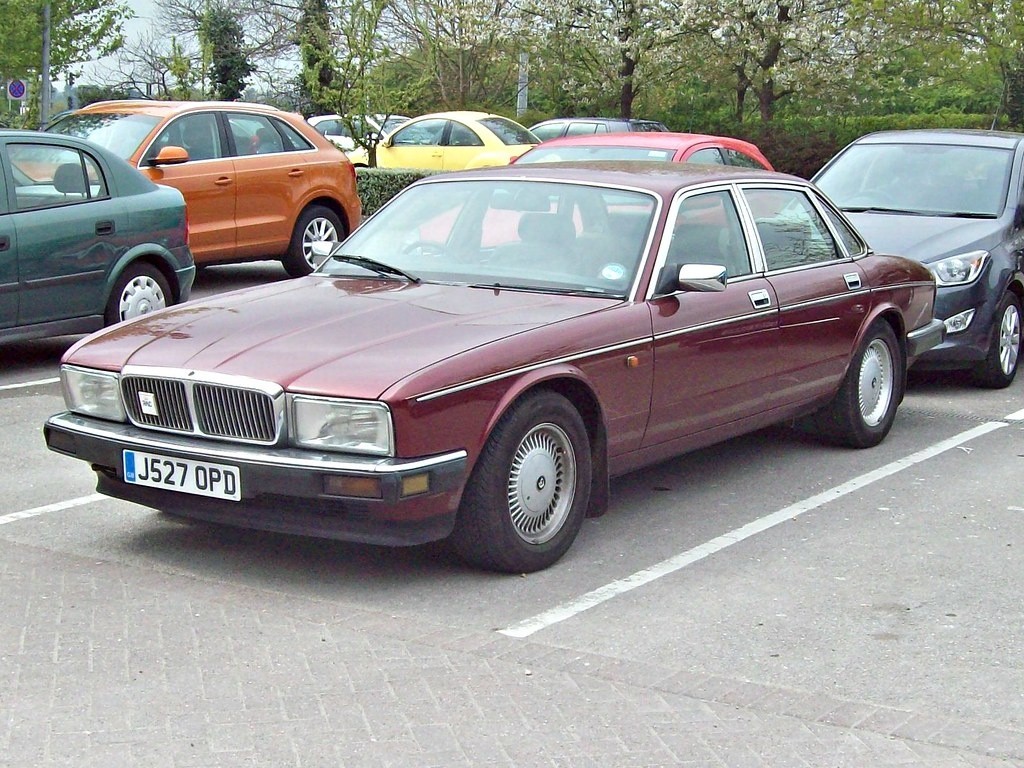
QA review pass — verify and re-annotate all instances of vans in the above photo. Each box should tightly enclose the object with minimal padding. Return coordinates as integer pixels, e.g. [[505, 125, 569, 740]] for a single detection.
[[526, 118, 669, 144]]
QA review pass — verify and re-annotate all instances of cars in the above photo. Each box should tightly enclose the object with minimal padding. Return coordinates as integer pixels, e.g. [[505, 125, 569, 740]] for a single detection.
[[782, 128, 1024, 389], [349, 109, 560, 184], [227, 118, 269, 151], [45, 162, 945, 577], [0, 128, 195, 353], [38, 99, 363, 277], [402, 131, 775, 256], [304, 113, 431, 169]]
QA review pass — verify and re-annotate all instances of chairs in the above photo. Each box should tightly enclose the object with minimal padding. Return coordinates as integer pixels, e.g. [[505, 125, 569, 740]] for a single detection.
[[490, 212, 581, 277], [53, 163, 88, 197], [183, 122, 213, 148], [453, 129, 473, 147], [674, 223, 734, 278]]
